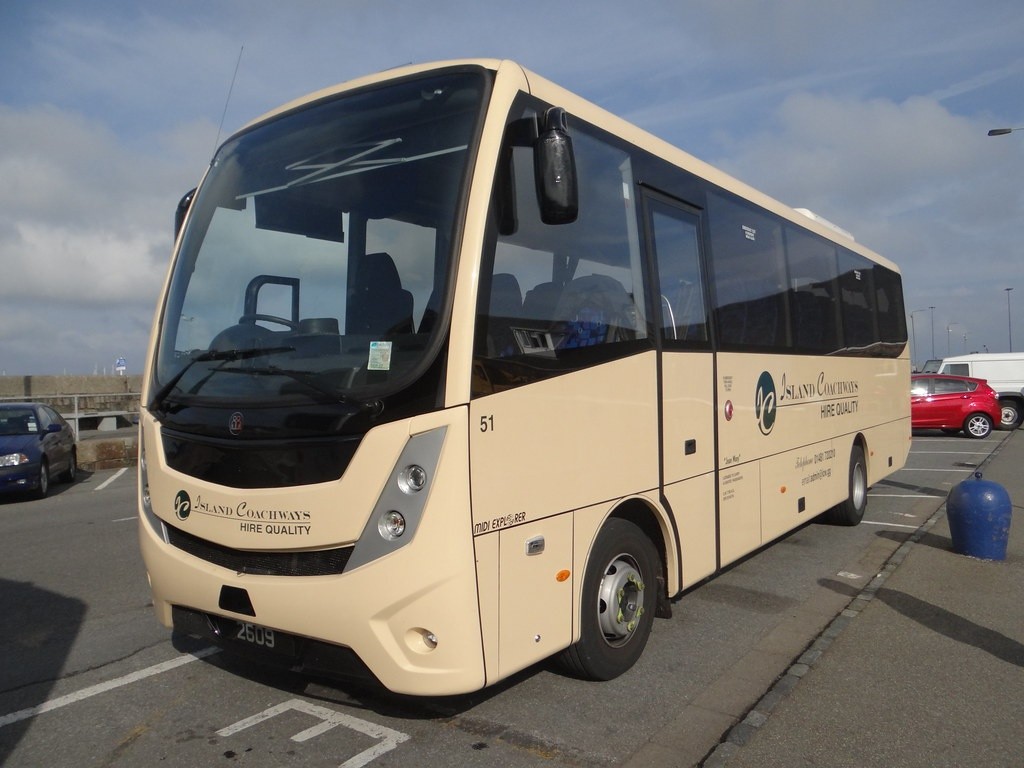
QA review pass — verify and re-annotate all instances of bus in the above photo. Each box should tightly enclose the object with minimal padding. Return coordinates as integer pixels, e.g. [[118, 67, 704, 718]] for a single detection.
[[129, 55, 914, 697]]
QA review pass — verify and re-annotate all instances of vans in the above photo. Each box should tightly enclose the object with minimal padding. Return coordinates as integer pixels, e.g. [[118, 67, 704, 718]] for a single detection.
[[919, 352, 1024, 431]]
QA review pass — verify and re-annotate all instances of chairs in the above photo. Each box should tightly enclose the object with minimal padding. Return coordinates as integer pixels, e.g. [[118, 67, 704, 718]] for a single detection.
[[492, 262, 897, 348], [7, 416, 29, 431], [343, 251, 414, 338]]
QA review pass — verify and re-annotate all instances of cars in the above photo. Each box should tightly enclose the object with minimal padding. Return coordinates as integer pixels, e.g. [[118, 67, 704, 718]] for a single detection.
[[0, 403, 78, 498], [911, 374, 1002, 439]]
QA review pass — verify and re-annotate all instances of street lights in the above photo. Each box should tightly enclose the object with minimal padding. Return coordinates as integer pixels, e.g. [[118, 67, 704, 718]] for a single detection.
[[1004, 288, 1014, 352], [929, 306, 937, 360], [947, 322, 958, 358], [910, 309, 925, 373]]
